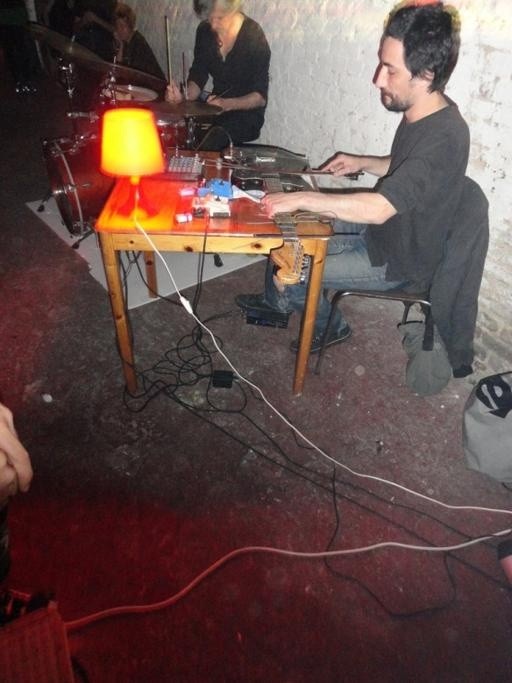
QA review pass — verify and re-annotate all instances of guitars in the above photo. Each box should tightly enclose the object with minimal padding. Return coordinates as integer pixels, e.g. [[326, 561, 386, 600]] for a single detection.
[[220, 144, 311, 286]]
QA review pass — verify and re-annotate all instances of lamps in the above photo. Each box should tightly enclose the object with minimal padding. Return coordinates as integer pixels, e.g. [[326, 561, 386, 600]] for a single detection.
[[102, 106, 170, 220]]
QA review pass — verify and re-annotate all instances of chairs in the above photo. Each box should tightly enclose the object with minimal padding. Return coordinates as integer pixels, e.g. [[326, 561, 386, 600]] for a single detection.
[[316, 173, 488, 377]]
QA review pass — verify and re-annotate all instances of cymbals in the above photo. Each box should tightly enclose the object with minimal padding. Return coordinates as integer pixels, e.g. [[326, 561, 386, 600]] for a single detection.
[[22, 21, 103, 63], [143, 97, 223, 116], [72, 55, 162, 82]]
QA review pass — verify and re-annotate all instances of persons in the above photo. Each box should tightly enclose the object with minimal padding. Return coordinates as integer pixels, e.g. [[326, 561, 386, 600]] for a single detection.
[[109, 4, 167, 104], [234, 3, 469, 352], [48, 0, 121, 90], [160, 0, 271, 151]]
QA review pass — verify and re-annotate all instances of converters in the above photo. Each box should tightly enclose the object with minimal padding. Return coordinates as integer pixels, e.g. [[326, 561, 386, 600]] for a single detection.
[[213, 369, 234, 389], [246, 309, 288, 330]]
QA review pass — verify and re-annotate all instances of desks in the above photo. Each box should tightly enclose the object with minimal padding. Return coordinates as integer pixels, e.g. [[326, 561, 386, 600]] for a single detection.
[[94, 149, 334, 396]]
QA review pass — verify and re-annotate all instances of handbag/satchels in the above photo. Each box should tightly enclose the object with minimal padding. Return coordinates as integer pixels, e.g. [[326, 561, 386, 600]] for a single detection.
[[462, 371, 512, 485]]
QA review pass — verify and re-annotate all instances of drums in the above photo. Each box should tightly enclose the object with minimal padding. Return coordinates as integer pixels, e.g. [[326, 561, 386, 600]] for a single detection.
[[66, 102, 101, 133], [100, 84, 158, 111], [42, 129, 115, 236], [156, 120, 188, 150]]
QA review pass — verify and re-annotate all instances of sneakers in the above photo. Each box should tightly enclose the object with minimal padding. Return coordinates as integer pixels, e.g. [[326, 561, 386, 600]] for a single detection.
[[289, 325, 353, 354], [1, 591, 50, 625], [235, 292, 294, 315]]
